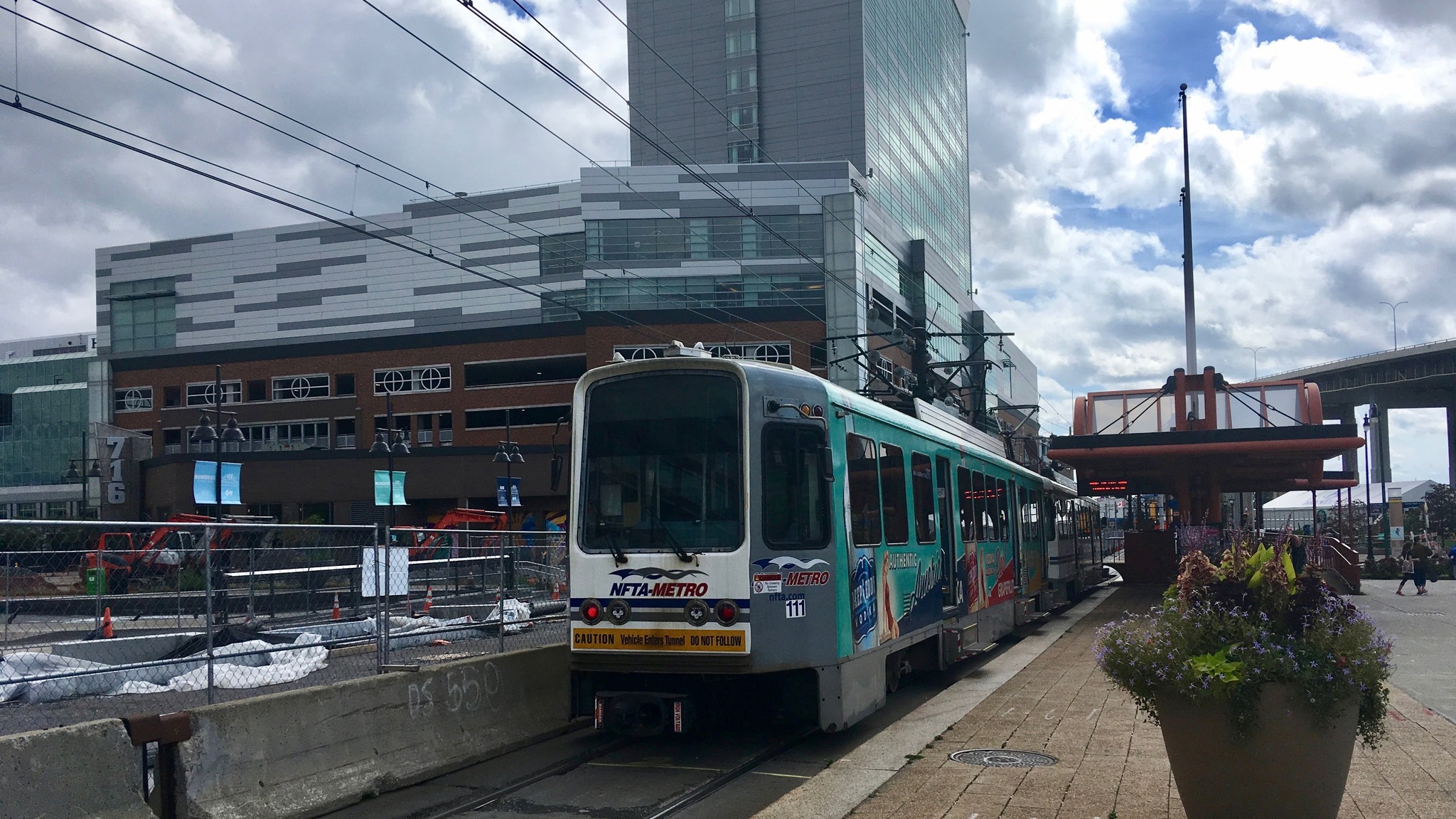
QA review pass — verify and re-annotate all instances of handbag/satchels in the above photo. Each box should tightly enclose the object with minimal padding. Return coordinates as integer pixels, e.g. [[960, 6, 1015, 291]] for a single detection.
[[1402, 550, 1414, 574]]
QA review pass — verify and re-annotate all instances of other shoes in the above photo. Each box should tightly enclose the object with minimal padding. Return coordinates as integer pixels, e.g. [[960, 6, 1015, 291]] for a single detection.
[[1422, 589, 1428, 593], [1395, 591, 1405, 596], [1417, 592, 1424, 595]]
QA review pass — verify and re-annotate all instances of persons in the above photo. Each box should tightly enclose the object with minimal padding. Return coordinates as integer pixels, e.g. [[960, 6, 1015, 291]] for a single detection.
[[1303, 524, 1311, 536], [1254, 518, 1257, 529], [1448, 543, 1456, 579], [1286, 535, 1307, 578], [1321, 527, 1333, 568], [15, 563, 19, 568], [1396, 535, 1433, 595]]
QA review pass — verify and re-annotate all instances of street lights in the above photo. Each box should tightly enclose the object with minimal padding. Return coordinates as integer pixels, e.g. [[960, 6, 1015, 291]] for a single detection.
[[190, 409, 248, 623], [1371, 402, 1389, 558], [369, 427, 411, 527], [63, 458, 107, 554], [493, 440, 525, 589], [1363, 414, 1375, 560]]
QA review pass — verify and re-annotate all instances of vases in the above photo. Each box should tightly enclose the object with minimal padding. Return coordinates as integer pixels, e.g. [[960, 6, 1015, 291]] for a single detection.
[[1155, 682, 1361, 819]]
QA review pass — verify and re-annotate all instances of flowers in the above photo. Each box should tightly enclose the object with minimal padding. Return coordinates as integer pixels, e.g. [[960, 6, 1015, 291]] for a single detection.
[[1092, 508, 1398, 753]]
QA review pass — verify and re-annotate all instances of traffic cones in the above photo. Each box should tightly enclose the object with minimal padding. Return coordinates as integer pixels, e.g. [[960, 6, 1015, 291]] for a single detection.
[[102, 608, 121, 640], [331, 592, 340, 619], [422, 587, 433, 614], [494, 586, 501, 604], [246, 608, 255, 623], [552, 581, 560, 600]]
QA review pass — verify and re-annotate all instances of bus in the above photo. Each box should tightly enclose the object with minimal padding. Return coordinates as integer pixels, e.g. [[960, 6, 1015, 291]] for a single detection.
[[551, 341, 1117, 735]]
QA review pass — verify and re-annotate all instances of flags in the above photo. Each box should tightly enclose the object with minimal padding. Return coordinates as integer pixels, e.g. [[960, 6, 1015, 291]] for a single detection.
[[1116, 500, 1125, 508], [1097, 498, 1105, 502]]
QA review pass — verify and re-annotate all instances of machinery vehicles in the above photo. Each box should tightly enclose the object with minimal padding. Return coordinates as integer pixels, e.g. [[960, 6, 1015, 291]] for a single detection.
[[78, 513, 238, 592], [143, 514, 278, 565], [378, 508, 508, 561]]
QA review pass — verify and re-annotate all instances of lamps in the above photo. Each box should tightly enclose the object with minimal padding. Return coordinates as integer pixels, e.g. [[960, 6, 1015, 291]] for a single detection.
[[971, 289, 977, 295], [967, 289, 971, 295], [866, 168, 873, 180]]
